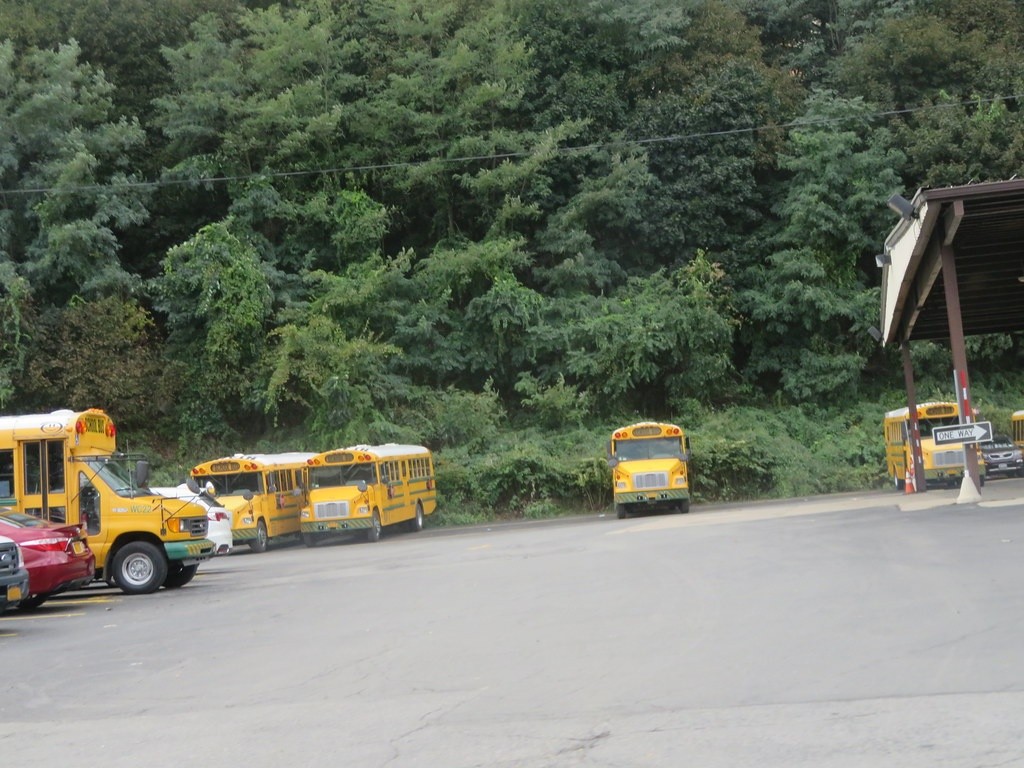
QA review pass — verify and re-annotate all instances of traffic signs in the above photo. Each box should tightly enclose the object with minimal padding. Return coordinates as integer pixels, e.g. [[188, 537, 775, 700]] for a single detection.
[[932, 419, 993, 446]]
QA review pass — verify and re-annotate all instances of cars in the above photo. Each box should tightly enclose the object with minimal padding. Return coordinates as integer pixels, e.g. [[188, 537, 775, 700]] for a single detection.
[[0, 505, 96, 610], [0, 535, 30, 613]]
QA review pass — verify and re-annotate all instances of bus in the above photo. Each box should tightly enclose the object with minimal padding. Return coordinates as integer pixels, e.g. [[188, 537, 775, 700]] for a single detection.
[[187, 452, 322, 552], [0, 405, 217, 595], [299, 442, 438, 547], [1012, 410, 1024, 450], [883, 400, 989, 490], [605, 421, 697, 515]]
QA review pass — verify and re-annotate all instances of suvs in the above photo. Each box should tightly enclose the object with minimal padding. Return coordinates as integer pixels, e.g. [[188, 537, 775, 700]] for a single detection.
[[147, 476, 233, 557], [979, 432, 1023, 482]]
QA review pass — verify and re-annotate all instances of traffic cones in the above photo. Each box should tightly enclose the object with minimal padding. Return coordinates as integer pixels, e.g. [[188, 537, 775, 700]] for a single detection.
[[902, 466, 917, 496]]
